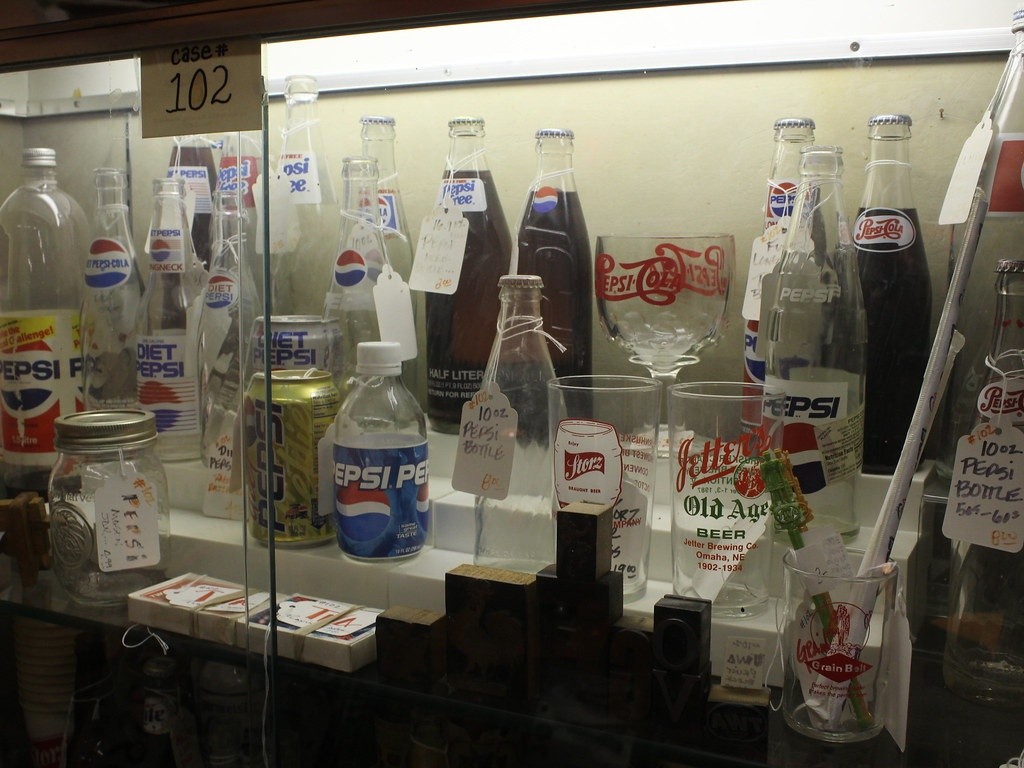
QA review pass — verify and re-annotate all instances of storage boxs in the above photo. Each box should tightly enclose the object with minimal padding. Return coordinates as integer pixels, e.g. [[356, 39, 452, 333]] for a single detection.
[[124, 571, 384, 672]]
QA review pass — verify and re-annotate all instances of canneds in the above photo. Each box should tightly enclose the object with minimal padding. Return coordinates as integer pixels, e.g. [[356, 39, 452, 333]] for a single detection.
[[249, 314, 345, 384], [242, 367, 341, 548]]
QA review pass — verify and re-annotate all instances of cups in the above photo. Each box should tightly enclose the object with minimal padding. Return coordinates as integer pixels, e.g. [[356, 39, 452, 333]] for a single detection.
[[781, 542, 898, 739], [546, 374, 663, 601], [13, 611, 74, 768], [666, 380, 784, 616]]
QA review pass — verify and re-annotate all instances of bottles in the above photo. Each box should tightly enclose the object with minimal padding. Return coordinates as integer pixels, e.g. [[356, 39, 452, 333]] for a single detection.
[[119, 660, 185, 768], [475, 274, 566, 570], [0, 72, 596, 471], [49, 406, 171, 610], [333, 340, 430, 564], [943, 259, 1024, 705], [742, 116, 934, 548]]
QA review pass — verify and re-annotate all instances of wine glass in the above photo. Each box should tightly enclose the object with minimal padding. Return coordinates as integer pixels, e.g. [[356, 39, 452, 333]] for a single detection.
[[595, 232, 738, 457]]
